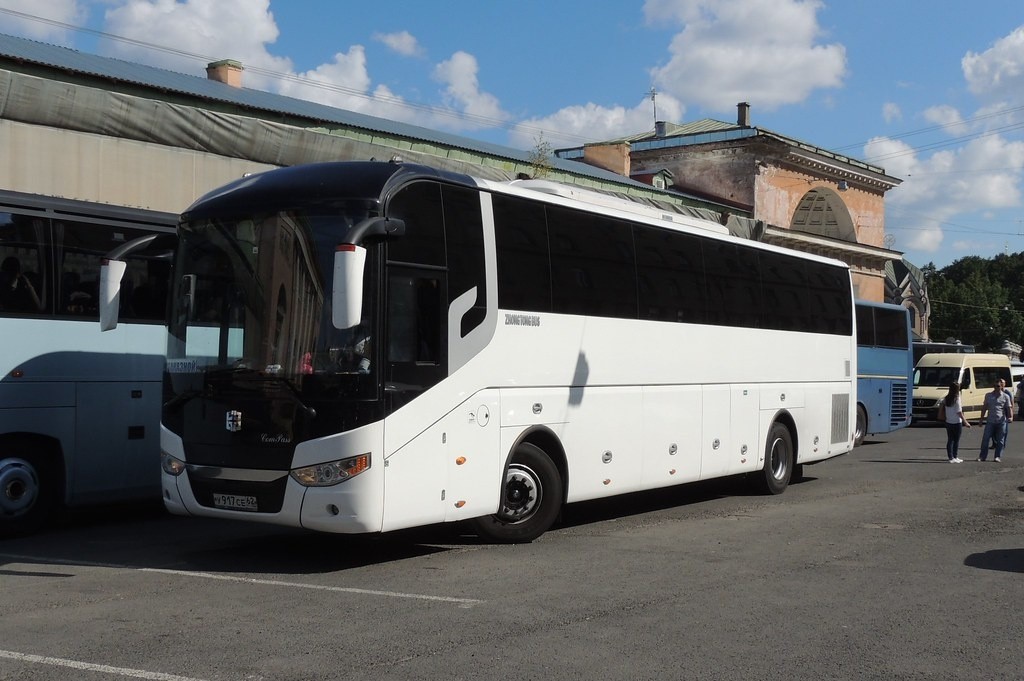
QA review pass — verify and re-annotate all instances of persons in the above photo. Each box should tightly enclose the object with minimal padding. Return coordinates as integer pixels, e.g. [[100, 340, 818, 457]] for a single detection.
[[977, 378, 1013, 462], [942, 380, 971, 463], [1015, 377, 1024, 419]]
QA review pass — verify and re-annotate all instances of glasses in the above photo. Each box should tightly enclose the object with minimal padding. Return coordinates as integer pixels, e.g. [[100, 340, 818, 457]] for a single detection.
[[992, 384, 1002, 387]]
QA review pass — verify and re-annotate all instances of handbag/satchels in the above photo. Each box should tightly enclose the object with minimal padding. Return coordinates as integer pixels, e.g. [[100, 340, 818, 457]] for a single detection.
[[938, 398, 947, 421]]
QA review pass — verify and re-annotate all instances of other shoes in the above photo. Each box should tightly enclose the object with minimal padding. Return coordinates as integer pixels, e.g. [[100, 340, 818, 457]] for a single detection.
[[994, 457, 1002, 462], [955, 458, 964, 463], [976, 457, 985, 461], [948, 458, 961, 464], [989, 445, 996, 449]]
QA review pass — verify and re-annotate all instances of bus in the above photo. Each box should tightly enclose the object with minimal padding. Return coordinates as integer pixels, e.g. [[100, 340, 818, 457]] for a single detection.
[[855, 299, 914, 449], [911, 341, 978, 367], [1, 191, 245, 534], [100, 156, 859, 541]]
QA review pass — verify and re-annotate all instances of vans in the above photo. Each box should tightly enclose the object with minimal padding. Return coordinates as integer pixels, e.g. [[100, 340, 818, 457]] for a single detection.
[[910, 353, 1016, 421], [1010, 363, 1024, 401]]
